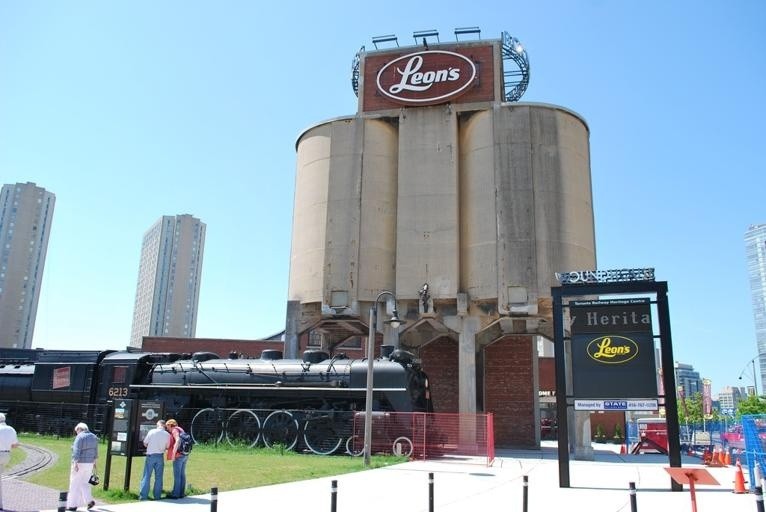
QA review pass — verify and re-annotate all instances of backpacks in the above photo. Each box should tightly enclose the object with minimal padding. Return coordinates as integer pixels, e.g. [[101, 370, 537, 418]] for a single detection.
[[176, 428, 192, 455]]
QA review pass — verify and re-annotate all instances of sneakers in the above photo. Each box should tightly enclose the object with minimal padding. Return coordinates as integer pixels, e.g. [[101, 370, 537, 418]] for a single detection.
[[87, 501, 95, 509]]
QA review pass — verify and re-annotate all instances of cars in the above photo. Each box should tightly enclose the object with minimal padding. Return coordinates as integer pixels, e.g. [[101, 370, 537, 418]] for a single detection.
[[719, 421, 766, 444], [539, 419, 558, 440], [679, 426, 719, 446]]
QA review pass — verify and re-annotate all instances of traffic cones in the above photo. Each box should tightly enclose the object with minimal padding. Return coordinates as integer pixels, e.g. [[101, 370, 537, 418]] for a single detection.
[[732, 464, 749, 495], [730, 457, 750, 484], [688, 446, 694, 456], [700, 445, 733, 468], [619, 444, 627, 455]]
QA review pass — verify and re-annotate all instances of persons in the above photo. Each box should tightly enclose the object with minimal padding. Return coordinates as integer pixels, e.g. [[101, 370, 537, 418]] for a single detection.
[[0, 412, 19, 511], [67, 421, 98, 512], [165, 419, 189, 498], [139, 419, 171, 501]]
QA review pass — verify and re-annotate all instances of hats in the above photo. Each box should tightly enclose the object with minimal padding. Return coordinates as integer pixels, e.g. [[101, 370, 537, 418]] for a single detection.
[[166, 418, 177, 425]]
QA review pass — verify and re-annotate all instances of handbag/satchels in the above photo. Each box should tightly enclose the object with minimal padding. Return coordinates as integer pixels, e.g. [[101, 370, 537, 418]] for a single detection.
[[89, 473, 99, 485]]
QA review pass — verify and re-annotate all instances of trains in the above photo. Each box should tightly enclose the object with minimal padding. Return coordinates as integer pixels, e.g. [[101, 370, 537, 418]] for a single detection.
[[0, 342, 449, 461]]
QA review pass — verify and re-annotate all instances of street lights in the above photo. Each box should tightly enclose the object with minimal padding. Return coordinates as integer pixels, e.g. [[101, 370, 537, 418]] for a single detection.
[[359, 289, 409, 466]]
